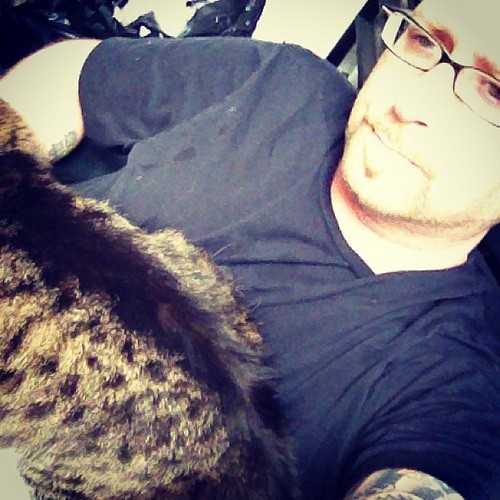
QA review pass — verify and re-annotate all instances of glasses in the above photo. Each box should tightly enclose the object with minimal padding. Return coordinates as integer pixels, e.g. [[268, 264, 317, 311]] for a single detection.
[[379, 3, 500, 128]]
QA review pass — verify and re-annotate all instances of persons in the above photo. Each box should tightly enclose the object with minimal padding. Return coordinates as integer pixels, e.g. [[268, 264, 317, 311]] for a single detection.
[[1, 1, 500, 500]]
